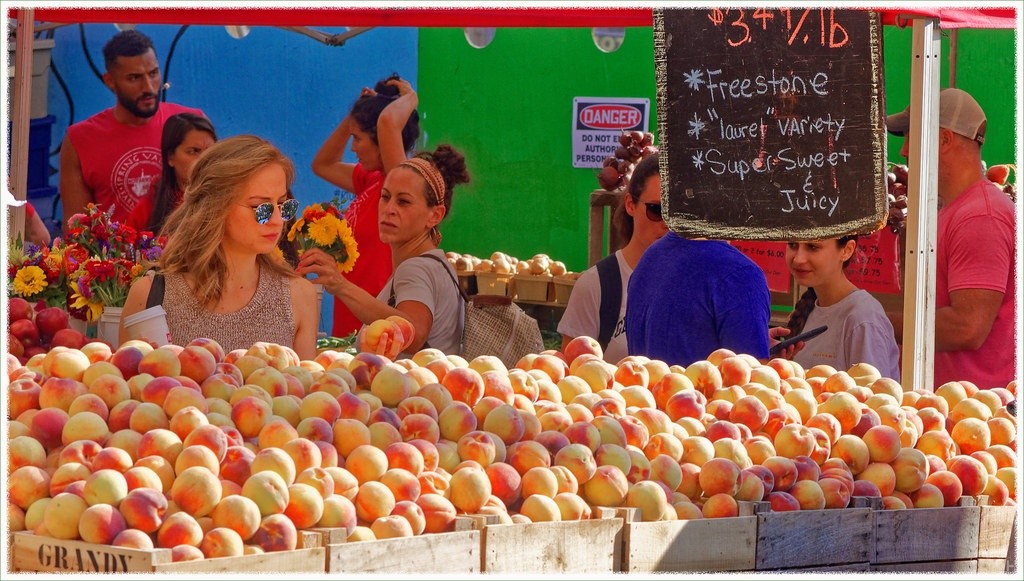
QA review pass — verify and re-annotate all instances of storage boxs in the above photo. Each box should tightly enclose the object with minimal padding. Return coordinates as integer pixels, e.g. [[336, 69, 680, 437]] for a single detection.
[[455, 271, 582, 304]]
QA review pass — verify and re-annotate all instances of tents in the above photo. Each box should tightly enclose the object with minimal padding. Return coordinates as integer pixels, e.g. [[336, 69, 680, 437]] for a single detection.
[[8, 7, 1014, 395]]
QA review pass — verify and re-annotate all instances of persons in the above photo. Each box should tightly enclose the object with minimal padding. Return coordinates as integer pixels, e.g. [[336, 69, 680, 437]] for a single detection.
[[885, 87, 1016, 394], [124, 112, 217, 251], [625, 233, 805, 366], [60, 29, 212, 247], [118, 136, 405, 360], [783, 234, 901, 385], [295, 144, 471, 361], [557, 153, 671, 367], [24, 202, 50, 248], [311, 72, 420, 338]]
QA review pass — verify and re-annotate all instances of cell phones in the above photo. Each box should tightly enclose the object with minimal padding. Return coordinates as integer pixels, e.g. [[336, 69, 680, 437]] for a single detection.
[[770, 325, 828, 357]]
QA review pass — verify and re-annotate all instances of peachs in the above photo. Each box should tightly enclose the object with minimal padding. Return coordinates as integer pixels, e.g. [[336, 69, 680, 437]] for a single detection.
[[365, 319, 395, 351], [385, 316, 414, 351], [7, 329, 1017, 564]]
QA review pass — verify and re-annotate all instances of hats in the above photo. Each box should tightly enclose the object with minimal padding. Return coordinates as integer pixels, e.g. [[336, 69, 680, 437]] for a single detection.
[[886, 87, 987, 146]]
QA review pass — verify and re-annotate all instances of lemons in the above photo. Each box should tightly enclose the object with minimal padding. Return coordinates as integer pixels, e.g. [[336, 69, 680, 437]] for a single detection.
[[442, 251, 580, 281]]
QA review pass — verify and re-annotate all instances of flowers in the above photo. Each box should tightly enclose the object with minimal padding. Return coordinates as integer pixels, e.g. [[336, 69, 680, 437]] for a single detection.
[[9, 202, 170, 324], [284, 197, 361, 274]]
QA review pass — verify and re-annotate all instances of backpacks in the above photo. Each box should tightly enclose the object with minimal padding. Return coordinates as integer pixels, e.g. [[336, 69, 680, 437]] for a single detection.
[[387, 253, 546, 369]]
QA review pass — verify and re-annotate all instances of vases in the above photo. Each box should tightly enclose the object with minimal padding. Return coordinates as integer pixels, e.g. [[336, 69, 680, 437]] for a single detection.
[[101, 306, 124, 350]]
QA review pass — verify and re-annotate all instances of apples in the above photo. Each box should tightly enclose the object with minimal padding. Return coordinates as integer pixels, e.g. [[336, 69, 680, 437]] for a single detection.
[[8, 296, 86, 364]]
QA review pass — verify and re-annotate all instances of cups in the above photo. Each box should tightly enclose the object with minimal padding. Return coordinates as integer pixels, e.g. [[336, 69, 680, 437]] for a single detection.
[[124, 305, 172, 347]]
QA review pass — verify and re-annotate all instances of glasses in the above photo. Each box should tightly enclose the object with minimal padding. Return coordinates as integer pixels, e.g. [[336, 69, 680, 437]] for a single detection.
[[637, 199, 663, 223], [231, 198, 300, 225]]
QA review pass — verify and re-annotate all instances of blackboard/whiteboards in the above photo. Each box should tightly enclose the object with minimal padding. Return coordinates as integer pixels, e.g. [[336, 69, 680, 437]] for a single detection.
[[655, 6, 888, 241]]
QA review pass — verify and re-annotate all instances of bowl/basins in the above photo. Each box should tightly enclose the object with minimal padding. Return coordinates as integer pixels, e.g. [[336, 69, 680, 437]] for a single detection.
[[455, 269, 576, 305]]
